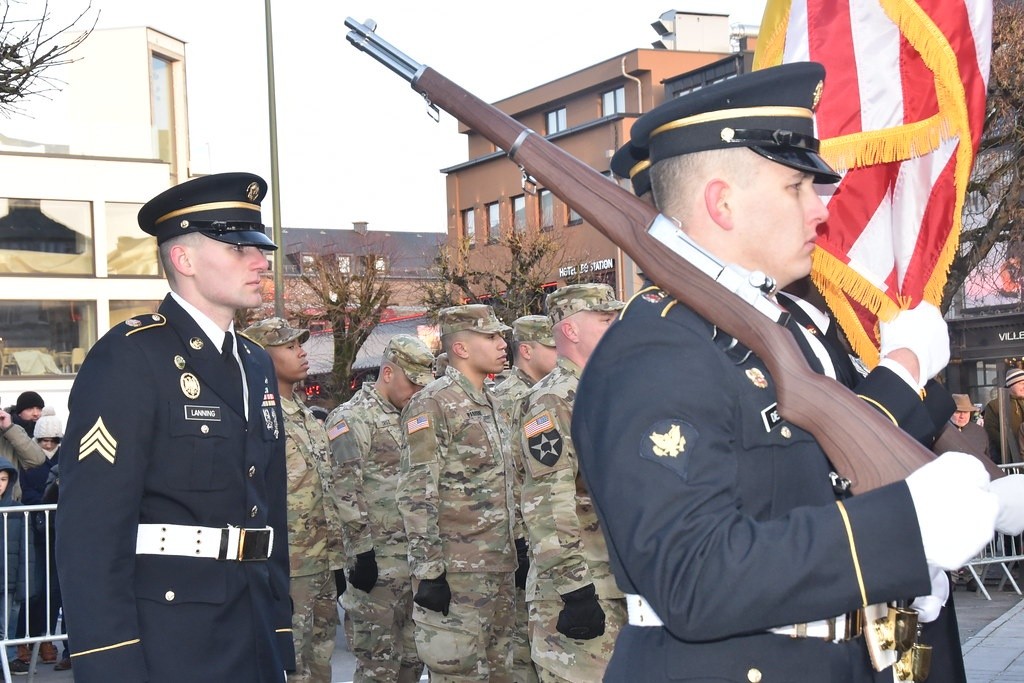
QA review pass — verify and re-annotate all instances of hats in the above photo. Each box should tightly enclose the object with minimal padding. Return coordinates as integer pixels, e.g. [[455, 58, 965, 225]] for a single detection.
[[609, 138, 651, 196], [16, 391, 44, 414], [34, 406, 63, 438], [630, 60, 842, 184], [952, 393, 979, 411], [242, 317, 310, 348], [1006, 369, 1024, 387], [139, 172, 278, 250], [383, 283, 627, 387]]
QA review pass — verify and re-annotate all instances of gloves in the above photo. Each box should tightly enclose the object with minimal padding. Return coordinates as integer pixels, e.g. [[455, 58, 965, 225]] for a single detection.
[[879, 298, 951, 387], [335, 569, 346, 598], [413, 569, 451, 616], [514, 537, 530, 590], [555, 583, 605, 640], [352, 548, 378, 593], [908, 571, 950, 623], [991, 474, 1024, 537], [906, 451, 995, 570]]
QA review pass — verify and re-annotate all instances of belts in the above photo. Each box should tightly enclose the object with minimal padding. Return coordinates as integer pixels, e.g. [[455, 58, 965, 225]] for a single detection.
[[135, 523, 274, 561], [625, 594, 863, 643]]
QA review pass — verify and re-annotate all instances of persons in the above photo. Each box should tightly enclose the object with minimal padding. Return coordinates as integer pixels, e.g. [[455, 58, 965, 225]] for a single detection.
[[511, 283, 627, 683], [572, 61, 1005, 683], [396, 304, 529, 683], [494, 315, 557, 413], [0, 391, 64, 675], [948, 394, 990, 459], [984, 368, 1024, 464], [55, 172, 297, 683], [242, 316, 348, 683], [322, 334, 436, 683]]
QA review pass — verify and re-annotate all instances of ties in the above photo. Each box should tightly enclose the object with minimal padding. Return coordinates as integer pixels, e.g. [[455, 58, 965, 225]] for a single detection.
[[220, 331, 245, 415]]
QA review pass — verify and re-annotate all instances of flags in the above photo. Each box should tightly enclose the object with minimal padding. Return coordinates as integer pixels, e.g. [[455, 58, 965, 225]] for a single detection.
[[752, 0, 993, 372]]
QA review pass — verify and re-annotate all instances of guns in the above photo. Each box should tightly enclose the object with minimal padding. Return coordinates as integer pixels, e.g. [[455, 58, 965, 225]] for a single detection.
[[339, 15, 937, 499]]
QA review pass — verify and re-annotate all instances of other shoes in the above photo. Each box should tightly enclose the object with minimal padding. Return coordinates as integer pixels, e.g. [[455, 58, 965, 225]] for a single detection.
[[9, 641, 71, 676]]
